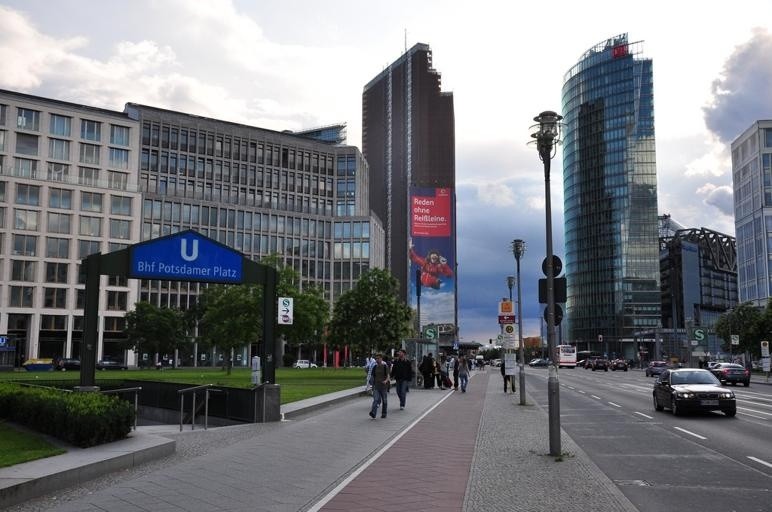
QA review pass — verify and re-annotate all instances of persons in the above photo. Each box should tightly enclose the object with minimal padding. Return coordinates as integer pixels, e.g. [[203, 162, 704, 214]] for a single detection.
[[501, 360, 516, 395], [364, 349, 486, 419], [409, 237, 453, 289]]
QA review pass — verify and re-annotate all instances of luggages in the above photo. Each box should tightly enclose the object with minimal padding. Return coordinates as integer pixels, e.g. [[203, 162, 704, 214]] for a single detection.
[[441, 370, 452, 388]]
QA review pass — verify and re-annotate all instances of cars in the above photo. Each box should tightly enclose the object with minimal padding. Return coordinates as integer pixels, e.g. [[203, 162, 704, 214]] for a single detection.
[[709, 362, 750, 386], [96, 359, 130, 371], [57, 356, 81, 369], [577, 358, 629, 373], [475, 353, 503, 368], [706, 360, 718, 368], [644, 360, 669, 376], [528, 356, 550, 368], [652, 368, 737, 417], [292, 359, 318, 369], [750, 360, 761, 370]]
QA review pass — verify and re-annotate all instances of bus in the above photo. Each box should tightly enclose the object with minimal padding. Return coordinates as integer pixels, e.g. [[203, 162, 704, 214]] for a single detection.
[[555, 343, 577, 369]]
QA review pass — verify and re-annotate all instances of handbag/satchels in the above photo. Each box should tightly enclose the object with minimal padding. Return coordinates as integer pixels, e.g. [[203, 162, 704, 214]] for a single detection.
[[369, 375, 374, 384]]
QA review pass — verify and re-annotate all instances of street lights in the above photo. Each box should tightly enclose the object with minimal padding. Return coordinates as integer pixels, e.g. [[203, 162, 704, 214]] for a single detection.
[[507, 238, 529, 404], [497, 273, 518, 394], [526, 110, 570, 455]]
